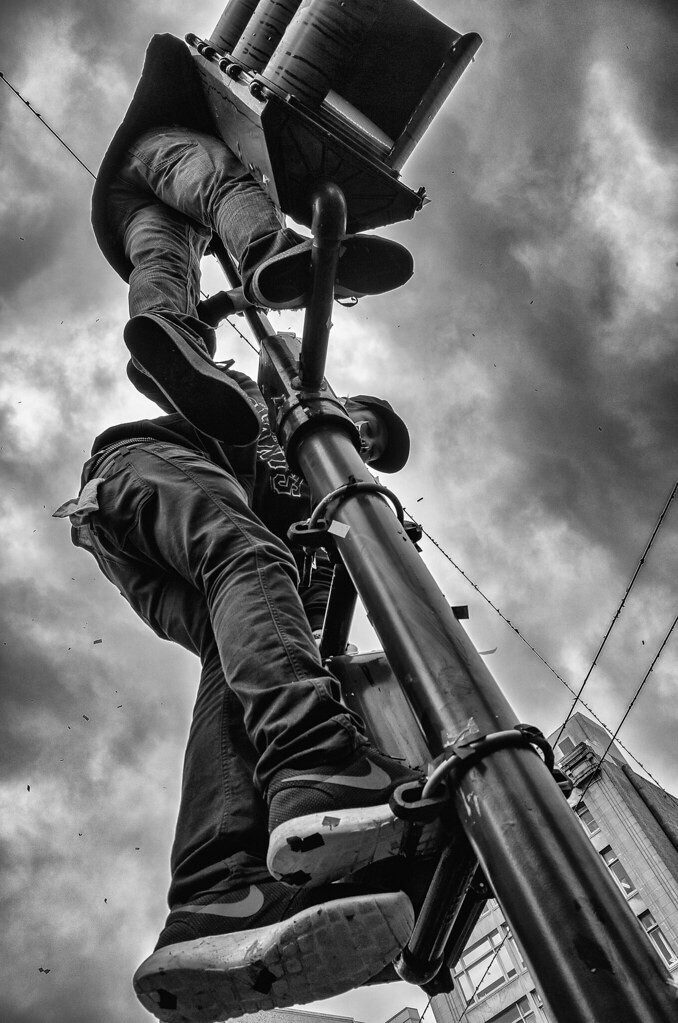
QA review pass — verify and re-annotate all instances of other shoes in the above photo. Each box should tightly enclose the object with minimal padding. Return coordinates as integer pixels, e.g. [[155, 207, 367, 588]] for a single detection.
[[250, 234, 414, 310], [125, 313, 262, 447]]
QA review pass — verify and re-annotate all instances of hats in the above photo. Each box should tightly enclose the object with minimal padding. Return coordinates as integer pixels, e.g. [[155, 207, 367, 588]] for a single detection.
[[342, 394, 412, 474]]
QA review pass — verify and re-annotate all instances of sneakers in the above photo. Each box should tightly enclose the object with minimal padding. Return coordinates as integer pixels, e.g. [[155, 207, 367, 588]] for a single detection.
[[133, 878, 415, 1023], [267, 747, 438, 887]]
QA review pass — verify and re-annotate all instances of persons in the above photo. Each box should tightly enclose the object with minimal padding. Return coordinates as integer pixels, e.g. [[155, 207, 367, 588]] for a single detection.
[[52, 395, 449, 1022], [90, 33, 414, 446]]
